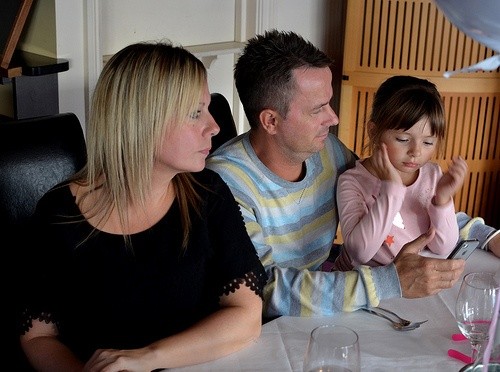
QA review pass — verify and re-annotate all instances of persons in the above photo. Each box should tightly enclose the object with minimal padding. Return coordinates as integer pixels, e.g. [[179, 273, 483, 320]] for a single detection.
[[204, 29, 499, 326], [4, 43, 269, 371], [329, 75, 469, 270]]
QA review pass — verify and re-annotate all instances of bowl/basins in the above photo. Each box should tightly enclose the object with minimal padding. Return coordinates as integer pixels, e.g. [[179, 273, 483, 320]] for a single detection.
[[459, 362, 500, 372]]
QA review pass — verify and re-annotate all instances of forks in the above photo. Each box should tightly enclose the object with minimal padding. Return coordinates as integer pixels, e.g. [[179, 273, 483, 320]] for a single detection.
[[377, 307, 429, 325]]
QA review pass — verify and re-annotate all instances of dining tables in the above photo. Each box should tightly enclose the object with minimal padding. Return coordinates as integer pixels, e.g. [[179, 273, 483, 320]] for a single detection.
[[150, 246, 500, 372]]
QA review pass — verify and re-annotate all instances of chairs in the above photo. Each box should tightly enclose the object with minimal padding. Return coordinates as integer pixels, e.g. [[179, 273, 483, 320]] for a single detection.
[[0, 113, 92, 232], [207, 92, 239, 160]]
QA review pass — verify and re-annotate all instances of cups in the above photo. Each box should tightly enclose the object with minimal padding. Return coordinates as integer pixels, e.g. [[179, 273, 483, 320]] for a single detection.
[[303, 324, 361, 372]]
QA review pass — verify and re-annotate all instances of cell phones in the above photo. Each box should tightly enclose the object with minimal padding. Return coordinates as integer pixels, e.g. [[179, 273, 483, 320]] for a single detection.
[[447, 239, 480, 265]]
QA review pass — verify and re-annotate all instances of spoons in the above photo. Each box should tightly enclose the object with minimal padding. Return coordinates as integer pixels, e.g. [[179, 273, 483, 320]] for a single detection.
[[360, 308, 416, 330]]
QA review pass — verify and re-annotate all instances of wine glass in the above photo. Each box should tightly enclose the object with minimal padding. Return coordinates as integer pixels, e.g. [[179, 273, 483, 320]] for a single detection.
[[457, 272, 499, 364]]
